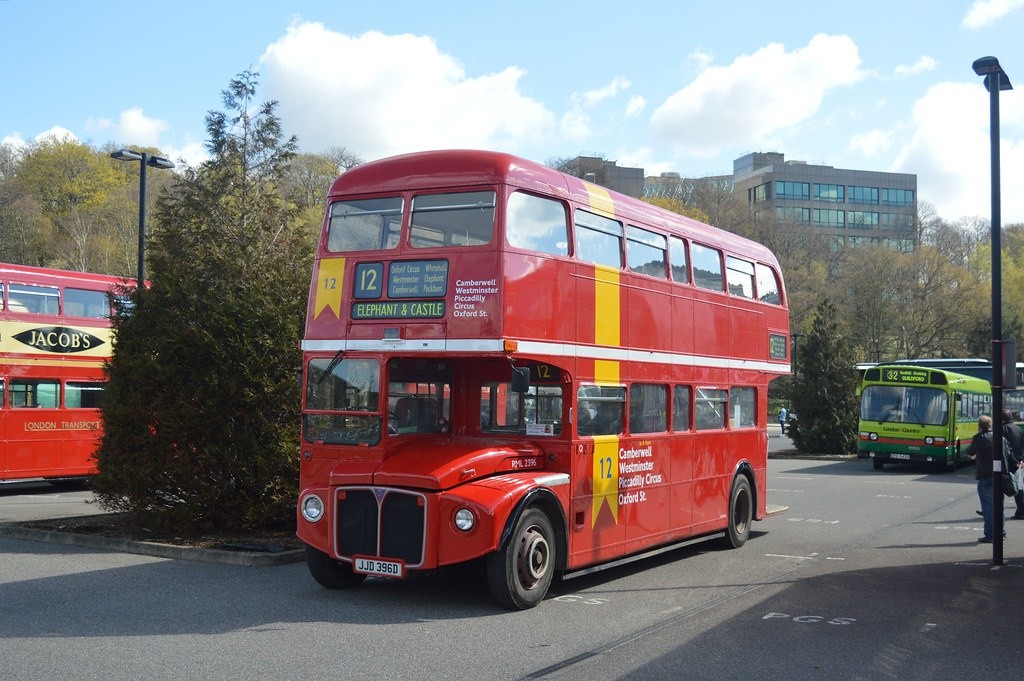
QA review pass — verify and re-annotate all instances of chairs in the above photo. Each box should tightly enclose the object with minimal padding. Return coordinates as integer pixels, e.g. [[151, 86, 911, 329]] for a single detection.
[[393, 396, 439, 432]]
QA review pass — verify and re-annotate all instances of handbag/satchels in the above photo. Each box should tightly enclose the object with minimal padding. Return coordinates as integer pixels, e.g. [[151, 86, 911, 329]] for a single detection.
[[1002, 472, 1017, 497], [1014, 460, 1024, 490]]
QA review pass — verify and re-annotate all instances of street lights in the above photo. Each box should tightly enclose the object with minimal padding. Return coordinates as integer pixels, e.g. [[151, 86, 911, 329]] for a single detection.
[[109, 149, 176, 288], [972, 57, 1013, 566]]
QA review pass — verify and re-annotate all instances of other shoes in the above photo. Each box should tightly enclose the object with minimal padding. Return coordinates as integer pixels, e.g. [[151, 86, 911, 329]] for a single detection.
[[976, 510, 983, 515], [1003, 533, 1007, 540], [978, 538, 994, 543], [779, 434, 785, 437], [1011, 515, 1024, 519]]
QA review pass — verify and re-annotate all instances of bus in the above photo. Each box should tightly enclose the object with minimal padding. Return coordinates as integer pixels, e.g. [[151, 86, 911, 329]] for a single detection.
[[396, 381, 601, 429], [857, 359, 1024, 420], [296, 149, 792, 610], [854, 365, 992, 469], [0, 263, 154, 483]]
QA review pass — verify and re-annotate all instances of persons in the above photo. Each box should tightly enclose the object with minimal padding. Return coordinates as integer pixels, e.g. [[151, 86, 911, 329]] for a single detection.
[[966, 416, 1024, 543], [778, 404, 786, 436], [976, 409, 1024, 519]]
